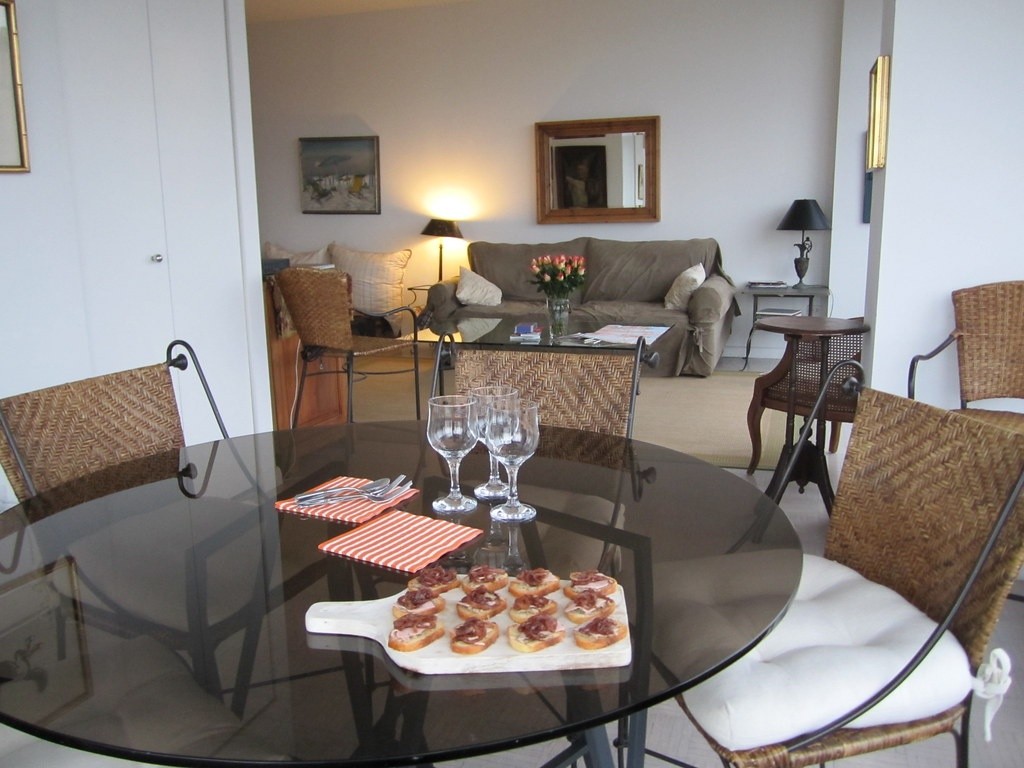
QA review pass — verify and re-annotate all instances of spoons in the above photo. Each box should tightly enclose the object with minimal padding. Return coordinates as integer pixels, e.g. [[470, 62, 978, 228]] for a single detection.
[[297, 477, 390, 501]]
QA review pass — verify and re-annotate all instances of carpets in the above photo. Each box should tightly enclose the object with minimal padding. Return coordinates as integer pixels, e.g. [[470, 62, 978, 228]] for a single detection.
[[345, 356, 806, 471]]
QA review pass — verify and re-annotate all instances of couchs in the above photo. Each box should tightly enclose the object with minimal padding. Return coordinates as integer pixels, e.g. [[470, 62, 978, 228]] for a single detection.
[[417, 236, 742, 378]]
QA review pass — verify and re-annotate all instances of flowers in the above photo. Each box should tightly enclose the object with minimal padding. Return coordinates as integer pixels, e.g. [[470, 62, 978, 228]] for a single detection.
[[526, 254, 586, 333]]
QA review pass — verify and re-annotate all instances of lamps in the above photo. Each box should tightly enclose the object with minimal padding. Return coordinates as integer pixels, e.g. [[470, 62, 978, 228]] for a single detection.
[[777, 199, 832, 291], [421, 219, 463, 282]]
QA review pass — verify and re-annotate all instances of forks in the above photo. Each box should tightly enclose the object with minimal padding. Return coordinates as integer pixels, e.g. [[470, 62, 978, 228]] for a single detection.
[[298, 474, 412, 507]]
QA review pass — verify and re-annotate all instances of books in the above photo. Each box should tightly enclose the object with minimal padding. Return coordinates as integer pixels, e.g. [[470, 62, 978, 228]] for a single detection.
[[509, 327, 544, 341]]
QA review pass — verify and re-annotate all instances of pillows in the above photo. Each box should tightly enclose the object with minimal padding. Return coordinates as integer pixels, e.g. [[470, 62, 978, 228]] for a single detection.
[[327, 242, 412, 336], [665, 263, 706, 313], [456, 265, 502, 306]]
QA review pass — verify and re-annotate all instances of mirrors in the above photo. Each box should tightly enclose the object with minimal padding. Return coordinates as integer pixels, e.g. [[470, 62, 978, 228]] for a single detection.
[[534, 115, 660, 225]]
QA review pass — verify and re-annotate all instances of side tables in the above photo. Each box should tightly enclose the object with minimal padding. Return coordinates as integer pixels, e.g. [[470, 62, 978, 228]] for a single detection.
[[408, 284, 431, 311], [739, 282, 830, 371]]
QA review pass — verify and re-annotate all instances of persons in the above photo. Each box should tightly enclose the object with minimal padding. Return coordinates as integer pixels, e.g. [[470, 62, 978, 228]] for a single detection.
[[565, 159, 606, 207]]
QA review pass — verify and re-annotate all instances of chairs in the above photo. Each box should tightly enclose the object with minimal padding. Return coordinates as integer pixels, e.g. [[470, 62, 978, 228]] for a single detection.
[[0, 333, 1024, 768], [746, 317, 864, 475], [276, 266, 427, 477], [906, 281, 1024, 603]]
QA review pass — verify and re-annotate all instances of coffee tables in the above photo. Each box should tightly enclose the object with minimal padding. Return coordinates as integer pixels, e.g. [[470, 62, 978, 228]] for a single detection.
[[395, 317, 677, 396]]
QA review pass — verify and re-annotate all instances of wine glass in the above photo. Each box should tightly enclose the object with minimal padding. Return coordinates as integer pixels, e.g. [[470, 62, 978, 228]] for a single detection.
[[485, 399, 539, 522], [468, 386, 519, 500], [426, 396, 479, 512]]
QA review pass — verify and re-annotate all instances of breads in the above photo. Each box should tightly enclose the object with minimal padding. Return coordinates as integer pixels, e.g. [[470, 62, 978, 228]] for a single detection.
[[387, 570, 628, 654]]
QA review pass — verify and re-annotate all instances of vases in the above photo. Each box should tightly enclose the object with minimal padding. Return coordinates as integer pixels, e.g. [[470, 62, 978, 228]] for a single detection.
[[548, 299, 570, 336]]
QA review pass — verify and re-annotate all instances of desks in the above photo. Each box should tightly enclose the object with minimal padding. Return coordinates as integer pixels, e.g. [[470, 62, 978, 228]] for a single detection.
[[754, 317, 871, 542], [0, 420, 802, 768]]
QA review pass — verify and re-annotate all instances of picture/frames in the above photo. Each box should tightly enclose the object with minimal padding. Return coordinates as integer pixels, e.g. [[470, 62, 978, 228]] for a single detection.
[[0, 556, 95, 727], [297, 135, 381, 214], [1, 0, 31, 174], [866, 56, 889, 172], [639, 164, 644, 200]]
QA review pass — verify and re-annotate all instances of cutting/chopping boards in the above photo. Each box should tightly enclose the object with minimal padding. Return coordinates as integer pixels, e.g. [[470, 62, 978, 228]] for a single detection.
[[305, 574, 632, 675]]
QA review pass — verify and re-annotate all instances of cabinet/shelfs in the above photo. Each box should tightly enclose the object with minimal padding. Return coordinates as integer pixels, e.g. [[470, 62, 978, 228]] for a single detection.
[[264, 277, 357, 479]]
